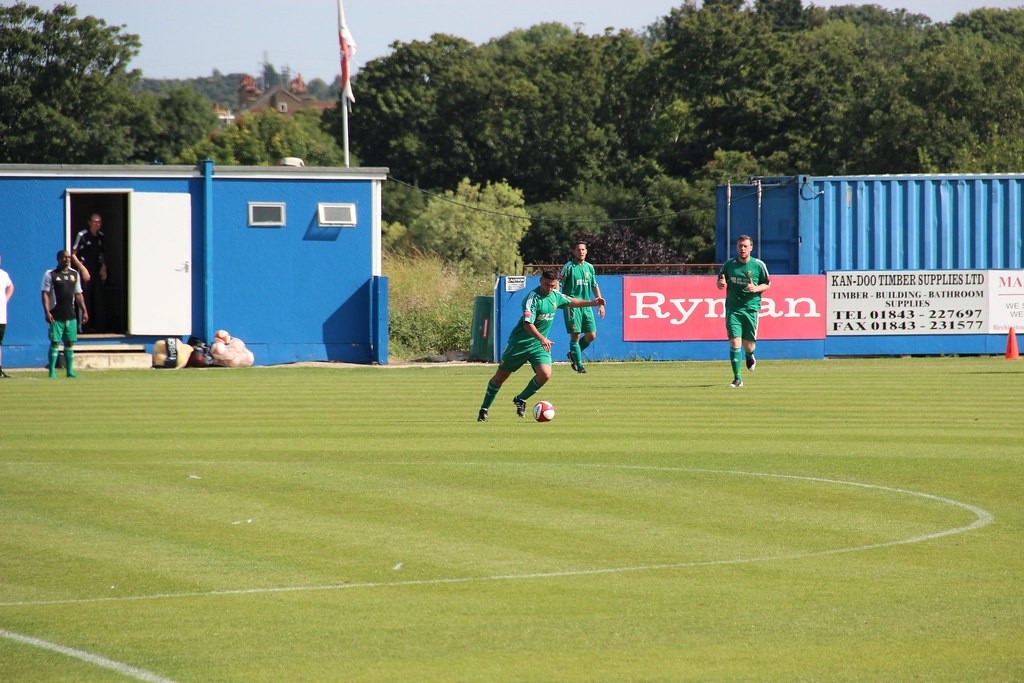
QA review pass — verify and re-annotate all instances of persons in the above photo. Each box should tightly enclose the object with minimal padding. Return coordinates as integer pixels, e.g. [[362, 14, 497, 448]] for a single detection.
[[41, 250, 89, 378], [716, 234, 772, 388], [477, 271, 607, 421], [71, 212, 106, 333], [0, 267, 14, 378], [554, 241, 606, 373]]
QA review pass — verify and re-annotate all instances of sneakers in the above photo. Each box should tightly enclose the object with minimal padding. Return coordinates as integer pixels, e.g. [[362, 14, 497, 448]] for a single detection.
[[513, 396, 526, 418], [0, 372, 10, 377], [578, 367, 585, 373], [746, 354, 756, 370], [730, 379, 743, 388], [68, 372, 78, 377], [50, 373, 56, 378], [478, 408, 488, 421], [567, 352, 577, 371]]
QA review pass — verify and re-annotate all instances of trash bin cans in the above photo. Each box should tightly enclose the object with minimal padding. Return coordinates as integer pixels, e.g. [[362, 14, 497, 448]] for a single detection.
[[469, 295, 495, 364]]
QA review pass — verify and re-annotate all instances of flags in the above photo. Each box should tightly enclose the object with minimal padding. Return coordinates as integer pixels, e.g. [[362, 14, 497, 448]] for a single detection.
[[337, 0, 356, 103]]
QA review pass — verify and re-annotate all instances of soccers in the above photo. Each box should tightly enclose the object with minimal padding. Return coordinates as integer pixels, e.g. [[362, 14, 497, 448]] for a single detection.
[[532, 400, 555, 423], [153, 329, 255, 369]]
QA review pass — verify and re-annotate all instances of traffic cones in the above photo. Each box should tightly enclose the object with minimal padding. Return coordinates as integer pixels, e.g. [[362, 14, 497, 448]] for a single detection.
[[1004, 327, 1024, 361]]
